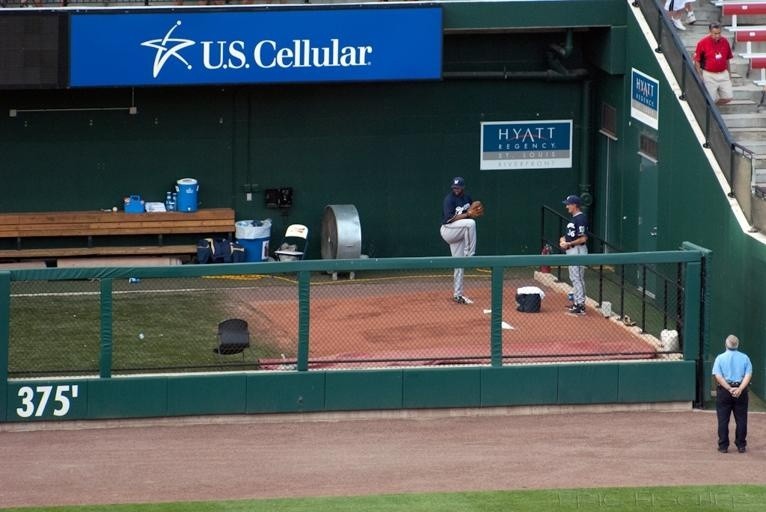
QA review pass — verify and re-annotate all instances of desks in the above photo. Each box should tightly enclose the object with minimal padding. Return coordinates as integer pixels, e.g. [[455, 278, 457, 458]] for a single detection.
[[0, 207, 237, 260]]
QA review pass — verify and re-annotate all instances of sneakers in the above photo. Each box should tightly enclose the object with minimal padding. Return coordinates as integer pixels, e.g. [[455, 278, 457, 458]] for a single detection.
[[454, 295, 474, 304], [564, 303, 586, 315]]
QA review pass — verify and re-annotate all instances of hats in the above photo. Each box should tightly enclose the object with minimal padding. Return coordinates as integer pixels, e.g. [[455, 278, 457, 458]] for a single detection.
[[451, 177, 466, 190], [562, 195, 581, 205]]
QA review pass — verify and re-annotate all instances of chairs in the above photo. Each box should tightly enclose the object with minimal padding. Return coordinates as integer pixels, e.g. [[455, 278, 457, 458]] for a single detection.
[[272, 222, 312, 282], [211, 316, 253, 371]]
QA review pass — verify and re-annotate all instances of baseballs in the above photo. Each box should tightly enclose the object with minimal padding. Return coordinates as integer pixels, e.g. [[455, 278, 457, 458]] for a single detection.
[[139, 333, 145, 340]]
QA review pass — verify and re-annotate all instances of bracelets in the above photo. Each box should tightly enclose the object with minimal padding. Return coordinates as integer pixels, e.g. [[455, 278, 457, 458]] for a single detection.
[[568, 242, 572, 246]]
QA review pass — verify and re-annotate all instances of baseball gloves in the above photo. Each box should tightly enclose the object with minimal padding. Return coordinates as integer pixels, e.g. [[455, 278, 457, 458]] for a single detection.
[[469, 200, 483, 216]]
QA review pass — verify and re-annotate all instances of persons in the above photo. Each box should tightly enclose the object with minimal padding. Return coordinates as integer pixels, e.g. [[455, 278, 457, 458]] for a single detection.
[[558, 196, 590, 318], [712, 333, 754, 453], [437, 176, 483, 306], [689, 21, 736, 108], [664, 0, 696, 31]]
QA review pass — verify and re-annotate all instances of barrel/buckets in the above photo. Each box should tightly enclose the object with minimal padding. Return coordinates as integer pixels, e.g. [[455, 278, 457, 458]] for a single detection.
[[175, 177, 200, 212]]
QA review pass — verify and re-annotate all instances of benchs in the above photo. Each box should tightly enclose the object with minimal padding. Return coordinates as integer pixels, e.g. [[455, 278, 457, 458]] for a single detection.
[[711, 0, 765, 88]]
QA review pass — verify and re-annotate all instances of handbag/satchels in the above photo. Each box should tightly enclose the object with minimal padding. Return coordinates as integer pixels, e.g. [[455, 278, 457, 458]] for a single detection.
[[189, 234, 246, 264]]
